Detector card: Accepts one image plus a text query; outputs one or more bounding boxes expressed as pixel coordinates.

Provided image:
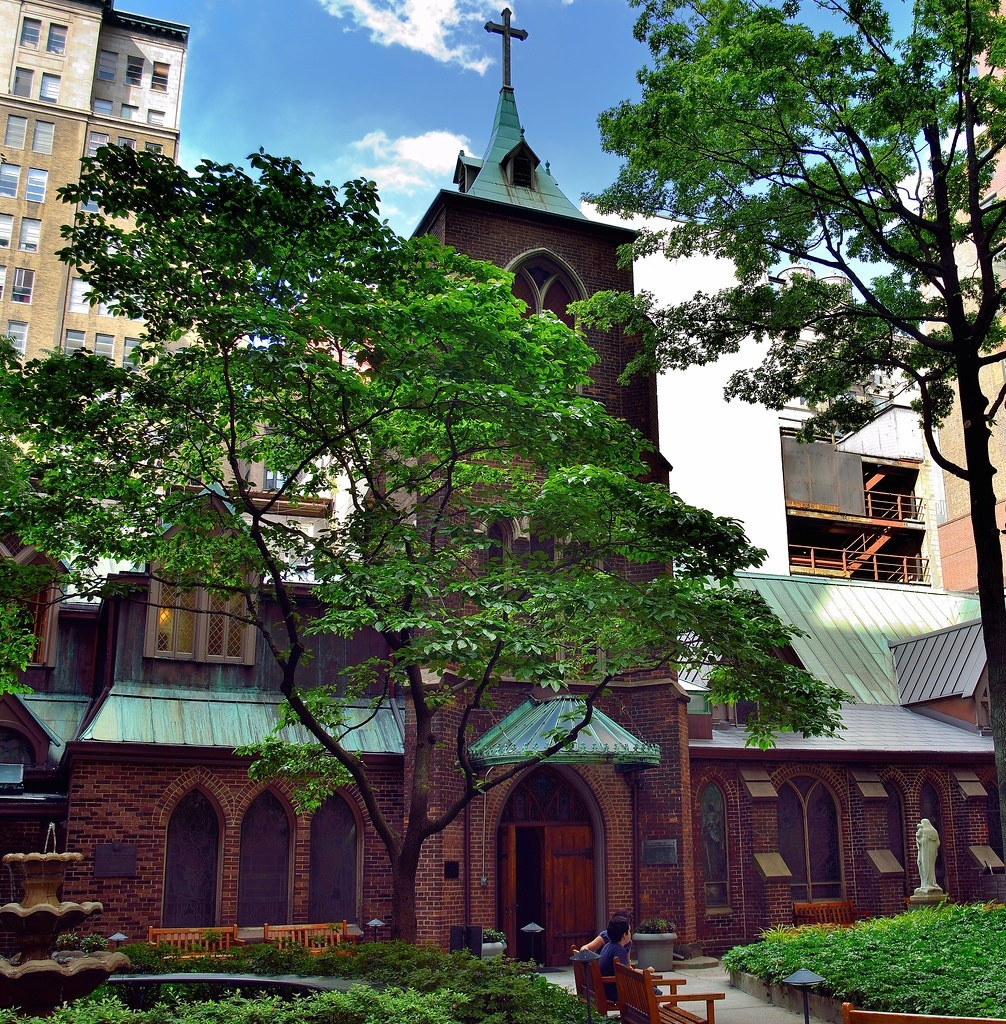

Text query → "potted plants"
[632,918,677,971]
[482,927,507,959]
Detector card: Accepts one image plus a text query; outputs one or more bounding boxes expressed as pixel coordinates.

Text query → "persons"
[581,910,657,987]
[599,915,663,1007]
[916,820,940,889]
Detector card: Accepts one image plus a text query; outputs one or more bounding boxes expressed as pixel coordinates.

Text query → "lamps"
[366,918,386,943]
[782,966,826,1024]
[520,922,544,986]
[0,763,24,795]
[108,932,128,949]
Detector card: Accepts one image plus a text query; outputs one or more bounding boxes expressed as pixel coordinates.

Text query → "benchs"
[842,1003,1006,1024]
[793,899,870,933]
[264,920,356,958]
[572,945,664,1016]
[150,924,245,960]
[613,956,725,1024]
[904,898,956,912]
